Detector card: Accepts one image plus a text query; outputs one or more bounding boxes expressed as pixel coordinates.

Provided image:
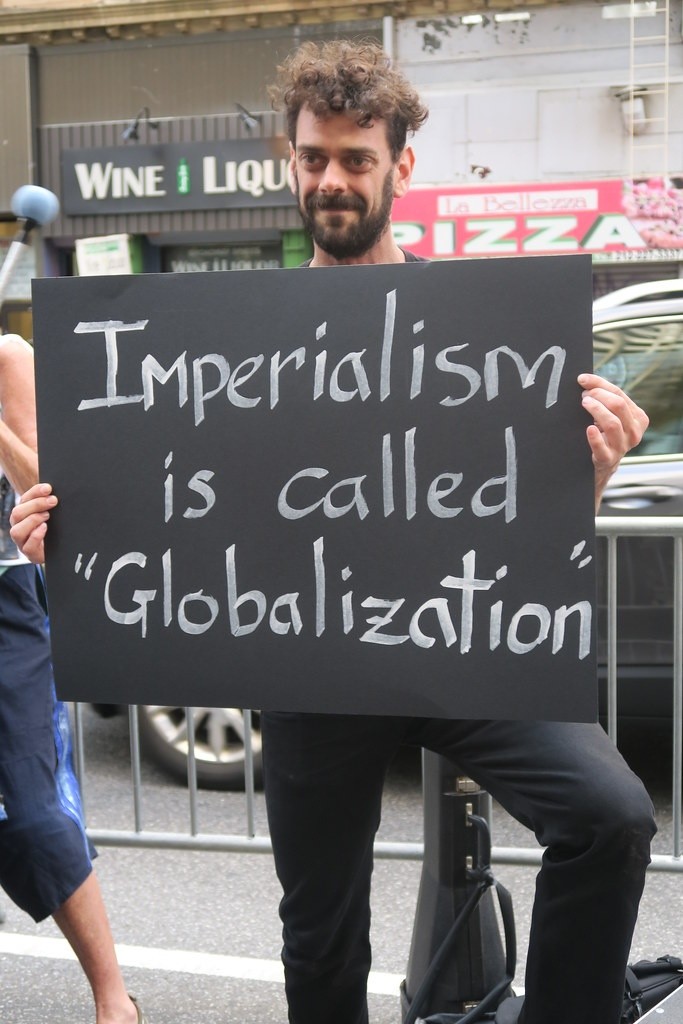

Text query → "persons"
[9,42,658,1024]
[0,333,143,1024]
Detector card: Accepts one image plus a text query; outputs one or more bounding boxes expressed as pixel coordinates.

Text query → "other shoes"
[129,995,148,1024]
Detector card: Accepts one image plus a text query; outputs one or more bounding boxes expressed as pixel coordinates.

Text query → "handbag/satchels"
[621,954,683,1024]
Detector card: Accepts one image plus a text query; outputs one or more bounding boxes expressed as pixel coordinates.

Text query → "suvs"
[90,279,683,791]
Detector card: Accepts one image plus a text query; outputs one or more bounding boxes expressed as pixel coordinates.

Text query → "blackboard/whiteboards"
[28,251,613,728]
